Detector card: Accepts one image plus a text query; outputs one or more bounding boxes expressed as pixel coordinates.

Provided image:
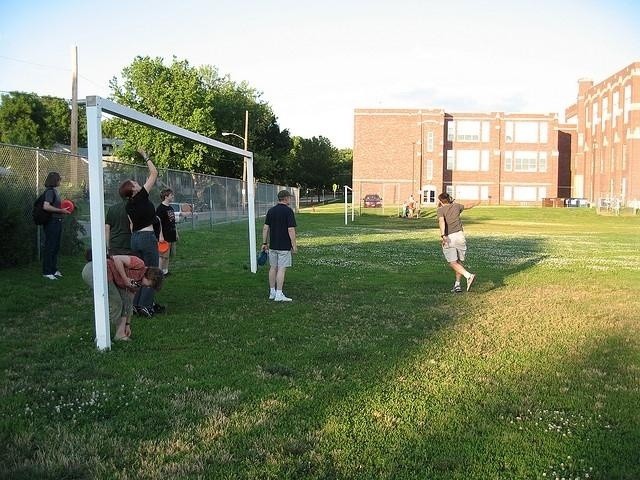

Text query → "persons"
[401,194,421,218]
[105,197,131,255]
[155,188,178,277]
[39,171,72,280]
[436,192,483,292]
[81,253,163,342]
[260,189,299,302]
[119,145,159,317]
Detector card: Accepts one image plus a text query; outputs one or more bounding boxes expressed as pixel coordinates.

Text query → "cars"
[362,193,383,209]
[169,202,199,224]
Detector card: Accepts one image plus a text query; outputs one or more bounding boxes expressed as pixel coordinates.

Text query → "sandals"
[114,335,133,342]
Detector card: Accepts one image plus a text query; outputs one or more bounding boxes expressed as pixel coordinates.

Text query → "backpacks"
[32,188,56,225]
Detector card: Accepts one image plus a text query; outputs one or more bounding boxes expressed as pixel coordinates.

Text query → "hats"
[277,190,295,197]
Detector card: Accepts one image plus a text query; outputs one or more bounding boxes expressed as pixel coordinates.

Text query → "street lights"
[220,130,247,214]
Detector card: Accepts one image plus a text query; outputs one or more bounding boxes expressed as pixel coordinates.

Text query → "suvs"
[179,198,209,212]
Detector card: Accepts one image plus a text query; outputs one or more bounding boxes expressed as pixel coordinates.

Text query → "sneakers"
[451,286,461,293]
[467,274,476,292]
[269,293,293,302]
[139,307,154,318]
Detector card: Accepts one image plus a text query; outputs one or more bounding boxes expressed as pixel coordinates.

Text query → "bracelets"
[441,234,446,238]
[261,242,269,245]
[125,322,130,324]
[143,157,151,164]
